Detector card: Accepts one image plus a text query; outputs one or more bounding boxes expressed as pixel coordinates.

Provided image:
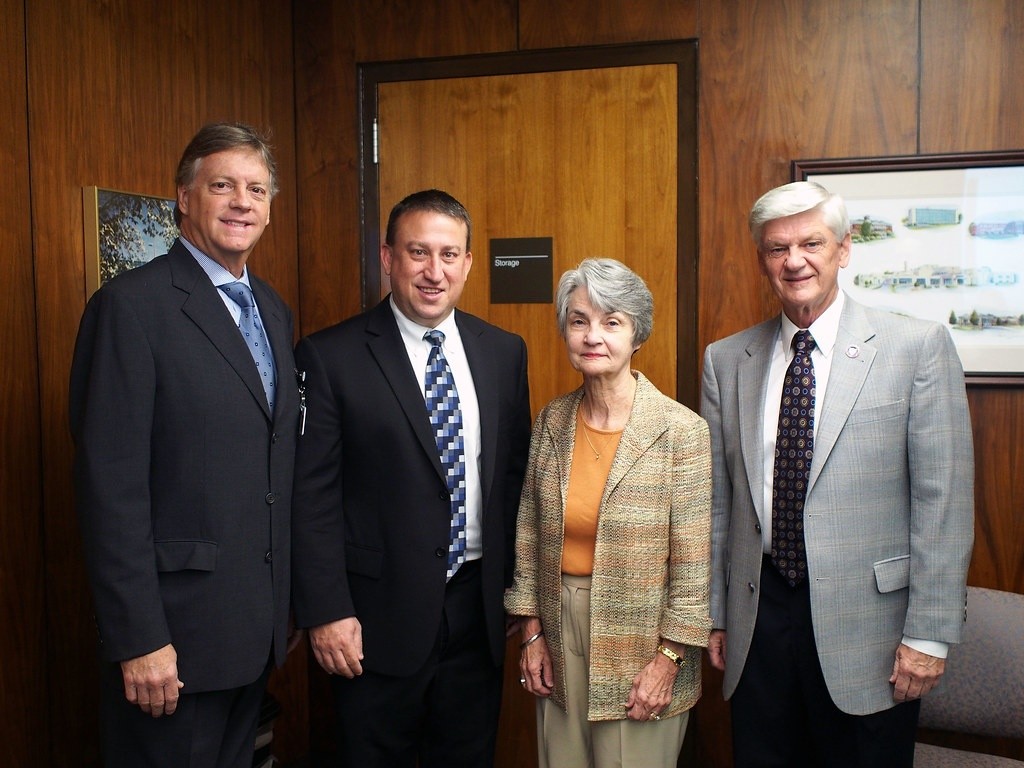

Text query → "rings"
[650,712,660,720]
[521,679,526,683]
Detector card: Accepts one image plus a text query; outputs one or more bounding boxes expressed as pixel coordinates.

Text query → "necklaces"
[582,392,631,461]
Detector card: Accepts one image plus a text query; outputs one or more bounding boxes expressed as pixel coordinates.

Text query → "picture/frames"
[791,150,1024,386]
[83,185,180,303]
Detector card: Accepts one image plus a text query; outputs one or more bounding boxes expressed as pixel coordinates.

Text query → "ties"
[772,329,820,590]
[221,280,278,415]
[423,330,467,586]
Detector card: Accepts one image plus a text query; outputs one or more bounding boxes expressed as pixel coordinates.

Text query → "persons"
[700,179,976,768]
[289,191,533,768]
[66,123,298,768]
[501,257,713,768]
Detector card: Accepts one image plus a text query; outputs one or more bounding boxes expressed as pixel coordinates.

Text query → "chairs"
[911,586,1024,768]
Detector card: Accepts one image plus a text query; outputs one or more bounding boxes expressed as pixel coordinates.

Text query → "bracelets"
[520,629,544,650]
[658,644,686,669]
